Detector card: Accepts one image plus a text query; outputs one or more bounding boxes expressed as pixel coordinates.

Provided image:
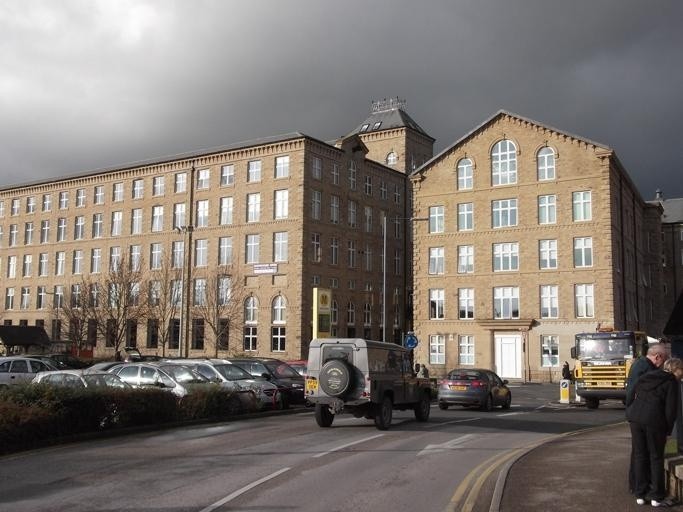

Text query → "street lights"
[174,225,194,357]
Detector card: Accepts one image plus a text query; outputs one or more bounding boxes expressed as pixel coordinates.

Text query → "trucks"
[570,327,662,410]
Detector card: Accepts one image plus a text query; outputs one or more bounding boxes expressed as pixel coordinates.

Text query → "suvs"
[303,338,435,431]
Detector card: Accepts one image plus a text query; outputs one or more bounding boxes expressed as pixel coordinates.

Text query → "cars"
[436,368,512,412]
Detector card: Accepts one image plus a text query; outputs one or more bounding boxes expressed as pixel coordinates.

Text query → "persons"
[562,361,570,378]
[626,345,668,494]
[416,364,429,379]
[625,358,683,508]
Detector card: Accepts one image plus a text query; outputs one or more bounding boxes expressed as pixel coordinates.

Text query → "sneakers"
[636,498,645,505]
[650,499,661,507]
